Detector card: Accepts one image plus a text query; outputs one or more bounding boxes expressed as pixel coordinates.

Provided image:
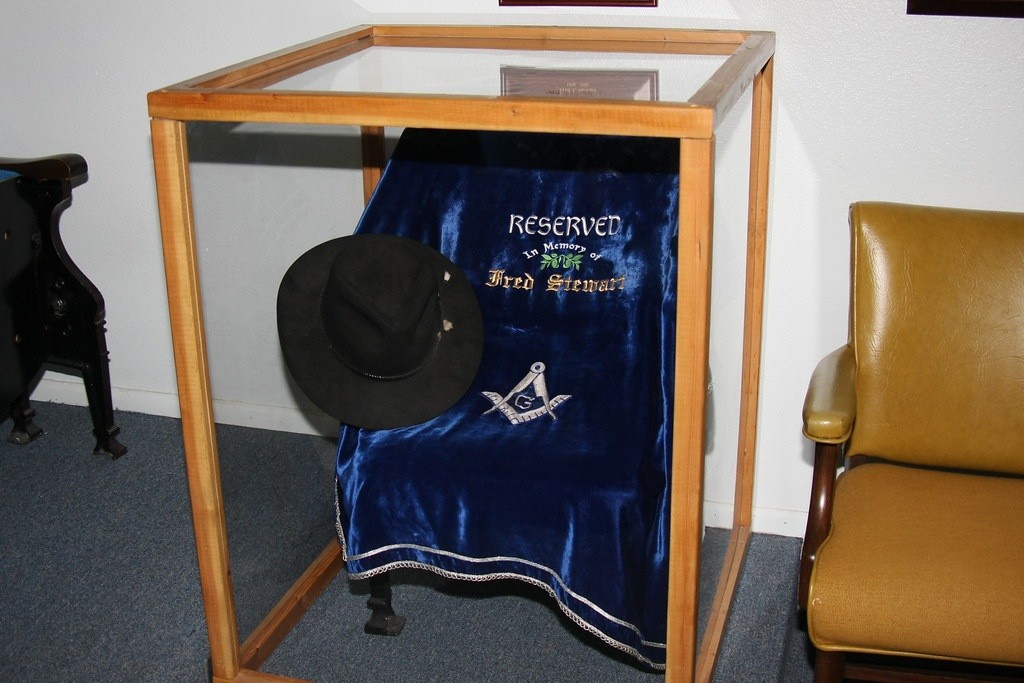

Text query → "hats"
[277,233,482,430]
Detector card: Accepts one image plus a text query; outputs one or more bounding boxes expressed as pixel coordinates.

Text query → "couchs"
[792,200,1024,683]
